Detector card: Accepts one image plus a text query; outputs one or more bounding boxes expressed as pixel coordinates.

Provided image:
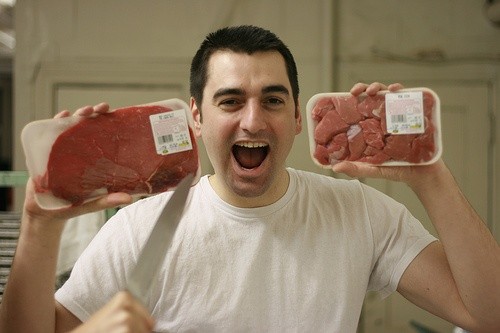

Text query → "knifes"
[124,171,195,305]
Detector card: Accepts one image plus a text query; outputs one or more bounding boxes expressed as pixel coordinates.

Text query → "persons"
[0,25,500,333]
[69,289,156,333]
